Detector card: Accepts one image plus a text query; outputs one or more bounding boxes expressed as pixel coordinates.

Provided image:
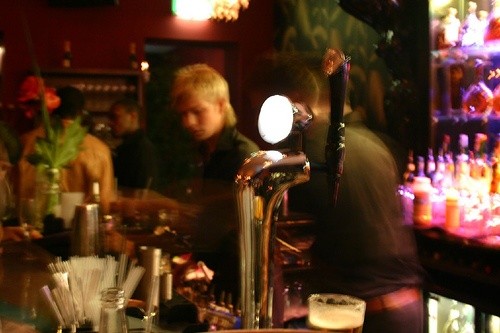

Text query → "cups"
[307,293,367,333]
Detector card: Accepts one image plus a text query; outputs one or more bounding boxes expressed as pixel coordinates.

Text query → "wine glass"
[18,197,46,264]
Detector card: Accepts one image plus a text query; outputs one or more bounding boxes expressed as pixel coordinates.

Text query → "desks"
[410,221,500,333]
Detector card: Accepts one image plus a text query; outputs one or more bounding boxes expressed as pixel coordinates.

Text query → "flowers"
[14,75,93,215]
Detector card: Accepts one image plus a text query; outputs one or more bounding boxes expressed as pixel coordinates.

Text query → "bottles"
[412,176,432,225]
[403,133,499,199]
[445,196,459,227]
[97,287,128,333]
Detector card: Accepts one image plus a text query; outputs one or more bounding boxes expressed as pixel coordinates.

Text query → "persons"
[93,98,167,192]
[110,63,260,308]
[249,47,429,333]
[15,85,115,227]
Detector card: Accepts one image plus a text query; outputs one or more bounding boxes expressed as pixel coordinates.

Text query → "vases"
[34,163,75,238]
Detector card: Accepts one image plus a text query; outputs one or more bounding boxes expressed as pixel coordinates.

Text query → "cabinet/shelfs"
[273,211,314,322]
[425,0,500,149]
[39,65,146,148]
